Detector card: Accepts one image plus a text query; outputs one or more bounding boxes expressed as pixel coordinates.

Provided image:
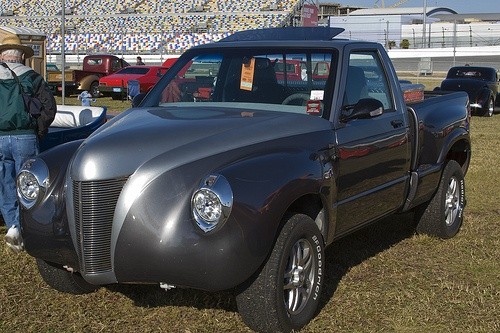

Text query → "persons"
[0,31,57,253]
[136,57,145,66]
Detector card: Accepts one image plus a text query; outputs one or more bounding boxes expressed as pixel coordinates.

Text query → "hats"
[0,33,35,58]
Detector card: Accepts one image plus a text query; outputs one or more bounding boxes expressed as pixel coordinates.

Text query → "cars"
[38,105,108,154]
[434,65,500,117]
[99,57,193,100]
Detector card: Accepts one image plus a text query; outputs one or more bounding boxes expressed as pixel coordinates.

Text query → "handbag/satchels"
[22,94,42,116]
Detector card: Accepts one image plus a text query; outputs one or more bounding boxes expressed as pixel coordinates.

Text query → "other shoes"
[5,225,23,251]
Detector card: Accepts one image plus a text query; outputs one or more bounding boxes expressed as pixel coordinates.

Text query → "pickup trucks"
[14,25,474,333]
[197,59,330,99]
[47,53,124,98]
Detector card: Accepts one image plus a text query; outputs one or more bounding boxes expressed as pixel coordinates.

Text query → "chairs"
[346,65,369,103]
[126,80,142,103]
[222,68,279,105]
